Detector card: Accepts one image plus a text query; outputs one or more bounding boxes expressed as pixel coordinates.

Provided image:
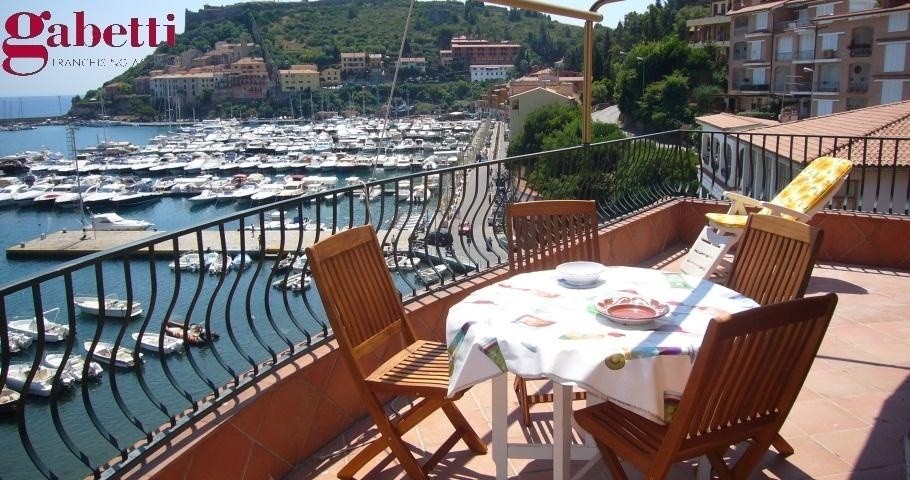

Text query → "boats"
[0,294,220,412]
[169,249,449,292]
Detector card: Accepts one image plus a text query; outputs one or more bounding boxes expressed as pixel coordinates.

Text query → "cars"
[479,119,522,253]
[457,220,472,236]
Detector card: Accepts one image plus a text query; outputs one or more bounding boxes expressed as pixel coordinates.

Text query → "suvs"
[424,230,453,247]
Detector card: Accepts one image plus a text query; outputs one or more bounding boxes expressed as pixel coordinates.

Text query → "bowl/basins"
[558,261,605,285]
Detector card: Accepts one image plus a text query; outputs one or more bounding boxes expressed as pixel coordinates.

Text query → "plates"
[595,296,670,324]
